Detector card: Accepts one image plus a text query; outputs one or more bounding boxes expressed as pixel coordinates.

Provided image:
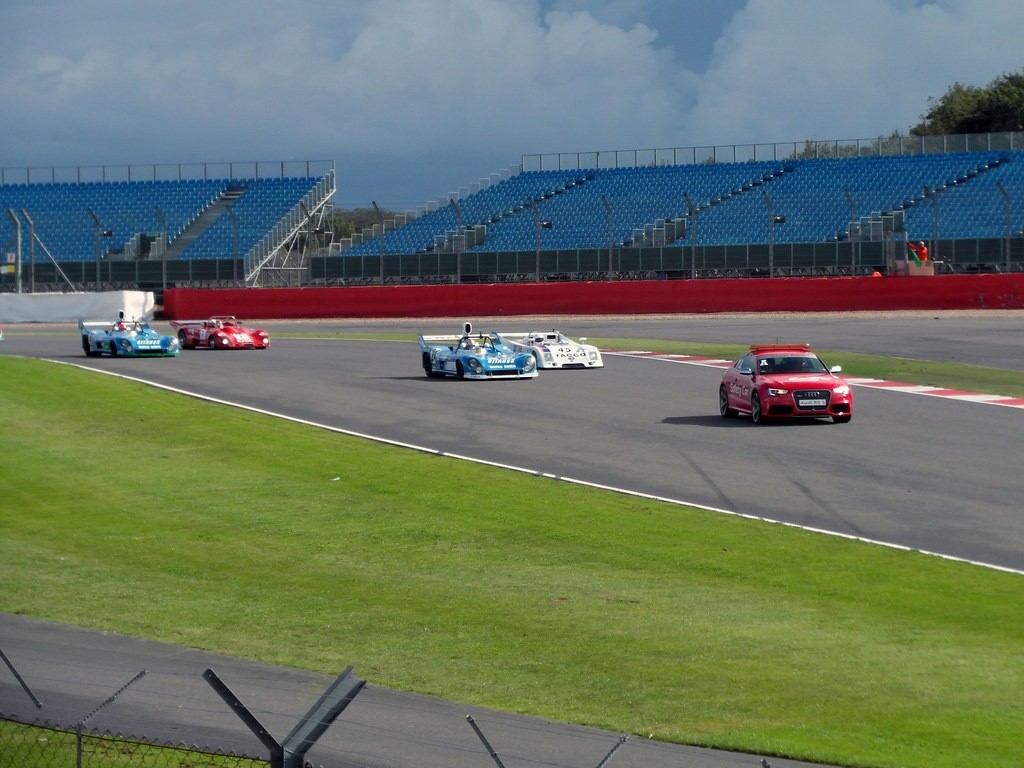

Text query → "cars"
[719,344,853,426]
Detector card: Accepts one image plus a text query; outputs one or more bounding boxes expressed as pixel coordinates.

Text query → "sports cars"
[169,315,270,350]
[417,322,539,382]
[488,327,605,370]
[78,310,179,358]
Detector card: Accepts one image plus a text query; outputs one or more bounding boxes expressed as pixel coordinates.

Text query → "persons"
[215,320,223,328]
[766,358,776,373]
[118,323,127,332]
[463,338,475,350]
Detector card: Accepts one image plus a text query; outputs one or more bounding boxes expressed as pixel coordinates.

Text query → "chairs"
[0,149,1024,266]
